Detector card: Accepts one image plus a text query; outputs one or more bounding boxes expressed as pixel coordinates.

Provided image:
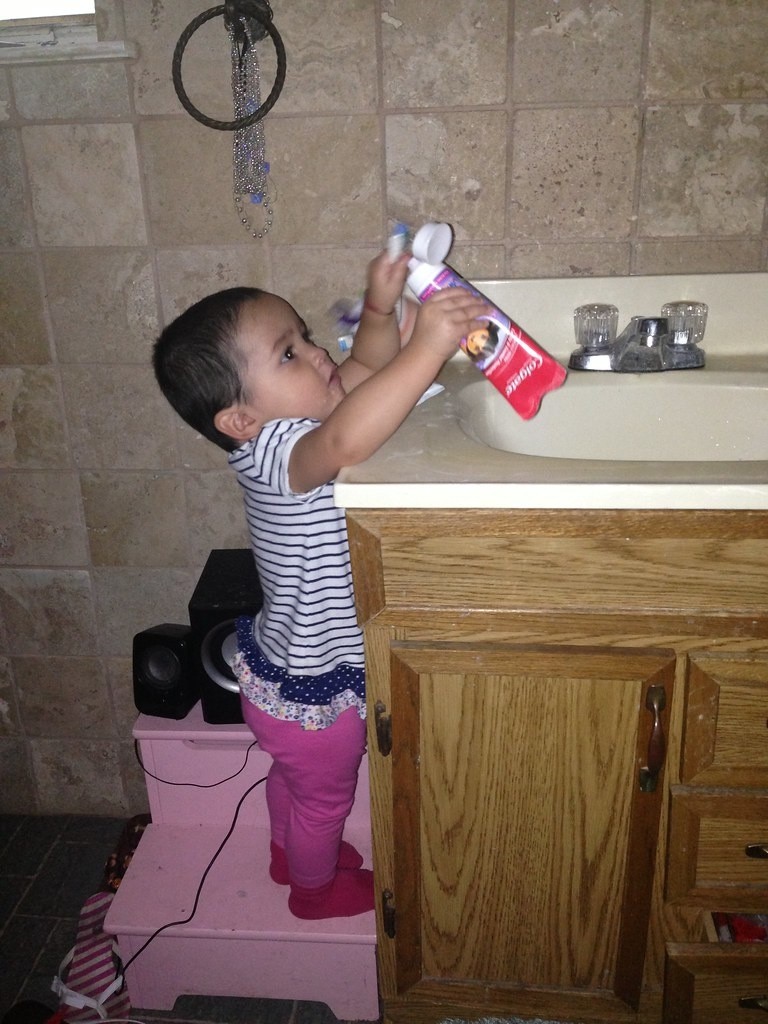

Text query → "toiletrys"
[405,220,571,421]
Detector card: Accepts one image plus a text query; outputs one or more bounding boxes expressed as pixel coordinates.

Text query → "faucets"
[611,315,670,372]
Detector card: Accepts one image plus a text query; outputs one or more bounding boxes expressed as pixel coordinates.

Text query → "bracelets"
[364,288,396,317]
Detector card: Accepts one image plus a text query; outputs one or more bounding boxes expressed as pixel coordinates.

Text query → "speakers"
[131,624,200,719]
[187,549,265,726]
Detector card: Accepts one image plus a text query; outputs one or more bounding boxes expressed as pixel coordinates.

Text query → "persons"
[153,250,488,920]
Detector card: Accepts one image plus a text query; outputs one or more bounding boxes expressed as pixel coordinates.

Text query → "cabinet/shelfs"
[346,504,768,1021]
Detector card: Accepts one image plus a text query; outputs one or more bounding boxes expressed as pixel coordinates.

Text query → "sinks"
[449,355,767,485]
